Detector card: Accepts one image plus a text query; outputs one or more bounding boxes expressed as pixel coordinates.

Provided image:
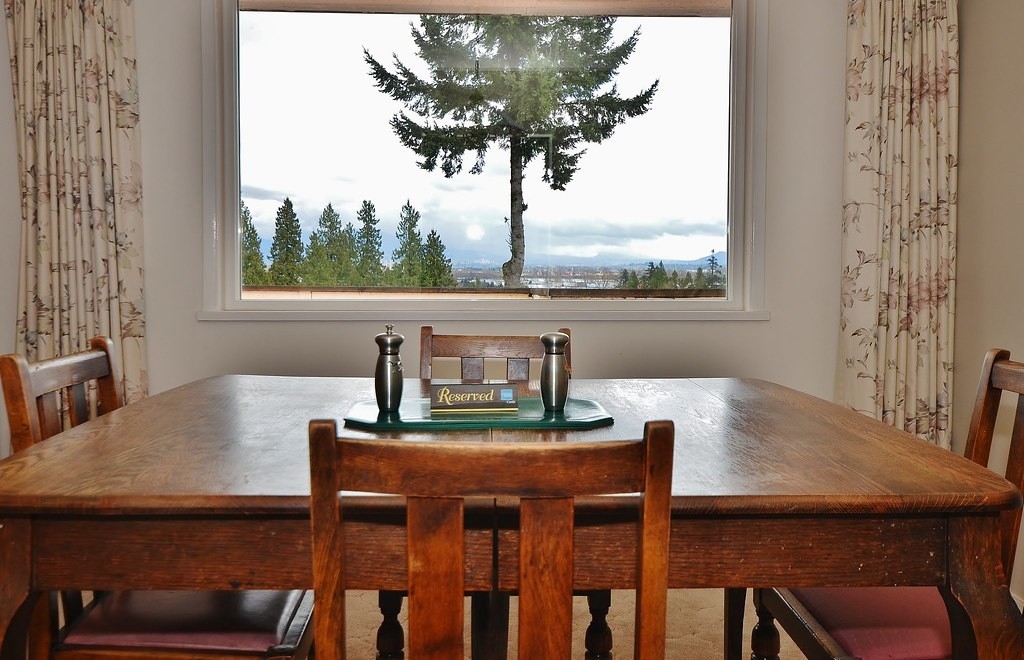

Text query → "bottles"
[541,333,569,415]
[373,325,405,414]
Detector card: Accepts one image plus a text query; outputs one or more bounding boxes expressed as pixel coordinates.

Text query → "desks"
[0,379,1024,660]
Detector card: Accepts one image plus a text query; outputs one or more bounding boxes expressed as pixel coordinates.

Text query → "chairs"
[309,325,675,660]
[750,346,1023,659]
[0,338,316,660]
